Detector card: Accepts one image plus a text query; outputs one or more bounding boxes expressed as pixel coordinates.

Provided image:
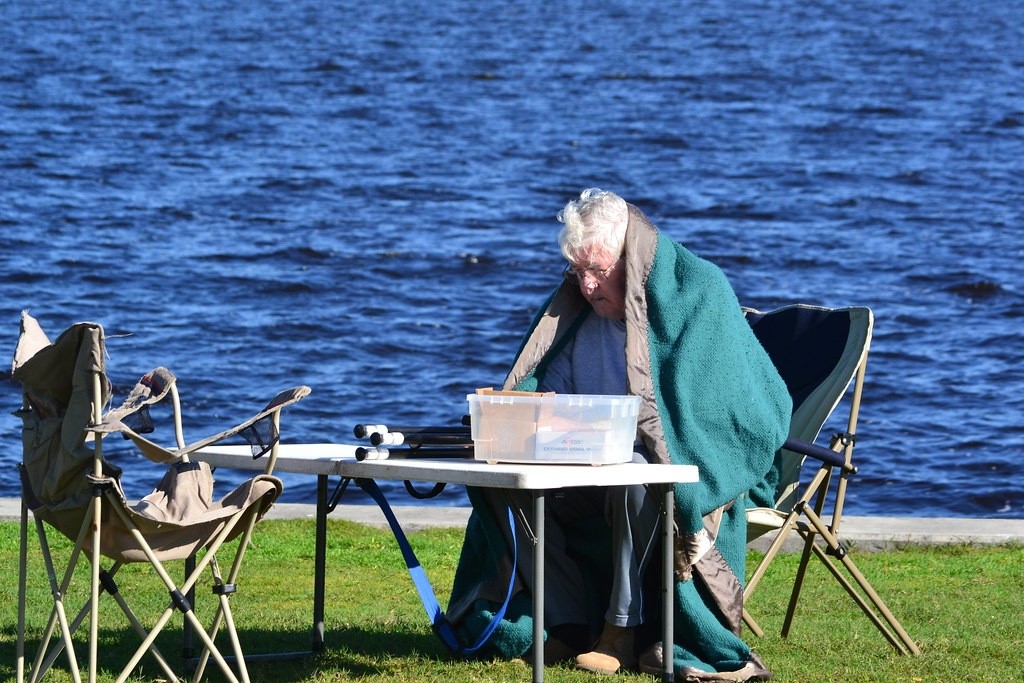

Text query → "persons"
[445,188,791,668]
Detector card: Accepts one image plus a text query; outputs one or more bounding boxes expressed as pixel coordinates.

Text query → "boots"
[574,623,634,675]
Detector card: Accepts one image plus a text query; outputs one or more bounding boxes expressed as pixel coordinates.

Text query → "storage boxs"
[467,392,644,466]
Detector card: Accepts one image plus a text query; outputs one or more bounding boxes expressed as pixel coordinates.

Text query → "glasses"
[562,252,625,283]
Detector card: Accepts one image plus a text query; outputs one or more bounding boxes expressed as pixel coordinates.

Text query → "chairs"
[738,303,924,658]
[10,308,312,683]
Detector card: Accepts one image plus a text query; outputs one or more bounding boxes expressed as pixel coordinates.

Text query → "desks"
[169,441,699,683]
